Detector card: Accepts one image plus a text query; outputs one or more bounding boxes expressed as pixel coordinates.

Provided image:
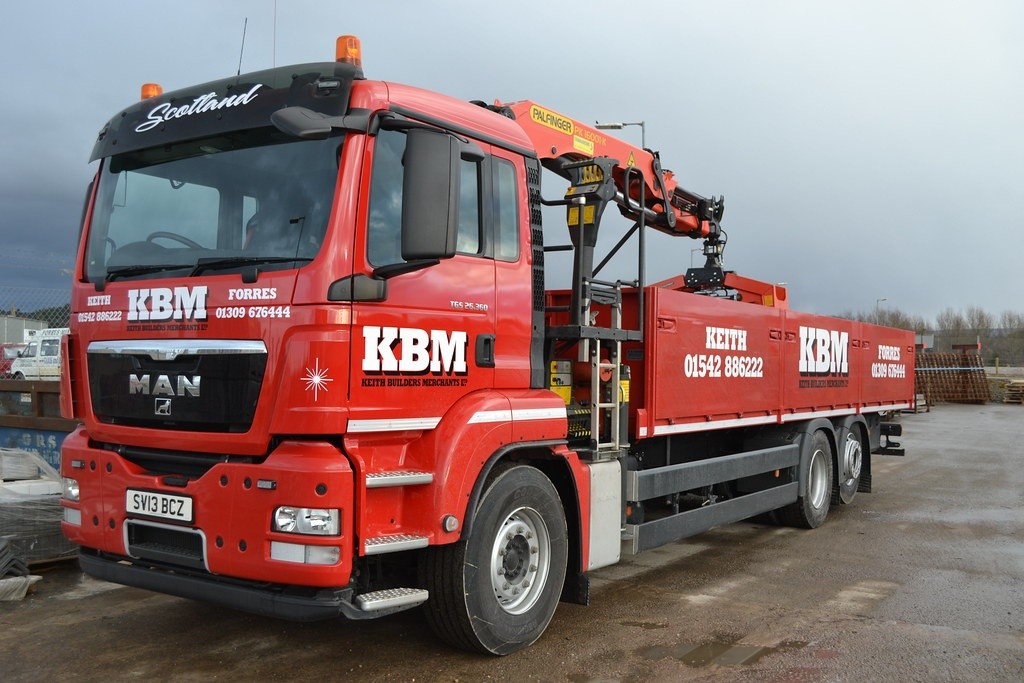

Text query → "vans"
[11,327,72,382]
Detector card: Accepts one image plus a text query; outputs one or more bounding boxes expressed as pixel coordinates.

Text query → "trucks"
[60,34,917,657]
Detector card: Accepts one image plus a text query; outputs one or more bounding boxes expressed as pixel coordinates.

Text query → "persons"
[241,185,320,259]
